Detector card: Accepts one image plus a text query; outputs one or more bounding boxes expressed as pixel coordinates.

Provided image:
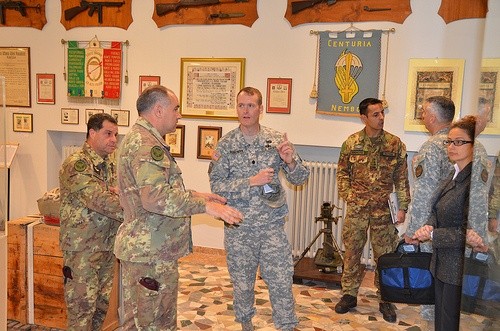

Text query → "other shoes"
[335,294,357,313]
[379,303,396,323]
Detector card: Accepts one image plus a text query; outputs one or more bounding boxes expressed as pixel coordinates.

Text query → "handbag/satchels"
[460,247,486,312]
[376,239,438,305]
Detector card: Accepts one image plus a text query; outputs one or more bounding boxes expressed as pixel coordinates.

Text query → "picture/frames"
[1,47,466,160]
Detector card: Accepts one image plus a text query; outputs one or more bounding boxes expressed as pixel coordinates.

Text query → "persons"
[208,87,310,331]
[412,115,477,331]
[59,113,124,331]
[404,96,462,321]
[113,84,245,331]
[465,97,500,331]
[335,98,411,322]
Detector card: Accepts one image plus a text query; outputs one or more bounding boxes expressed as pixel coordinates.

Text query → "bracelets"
[431,230,433,241]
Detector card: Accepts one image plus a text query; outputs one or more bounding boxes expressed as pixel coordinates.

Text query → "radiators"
[292,160,348,257]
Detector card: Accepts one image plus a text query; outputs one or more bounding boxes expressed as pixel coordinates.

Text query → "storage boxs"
[7,215,122,331]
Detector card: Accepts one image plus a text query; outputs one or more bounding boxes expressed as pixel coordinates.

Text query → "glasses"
[443,140,472,146]
